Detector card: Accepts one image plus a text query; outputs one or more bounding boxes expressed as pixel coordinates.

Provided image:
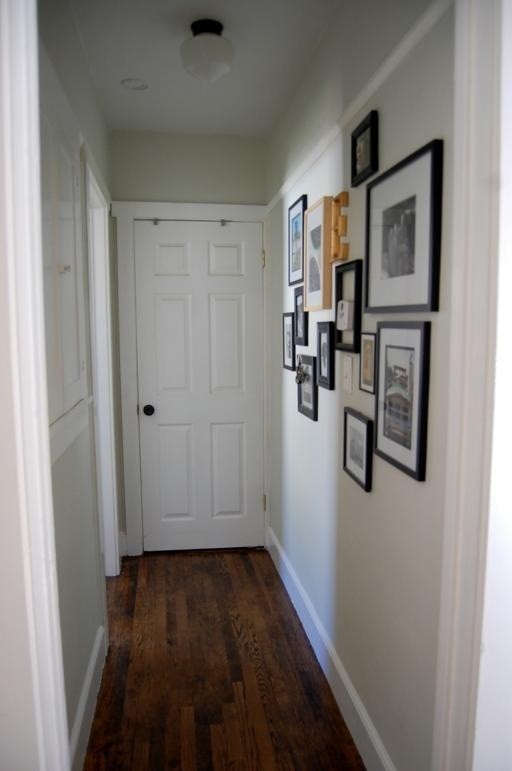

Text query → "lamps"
[179,15,236,85]
